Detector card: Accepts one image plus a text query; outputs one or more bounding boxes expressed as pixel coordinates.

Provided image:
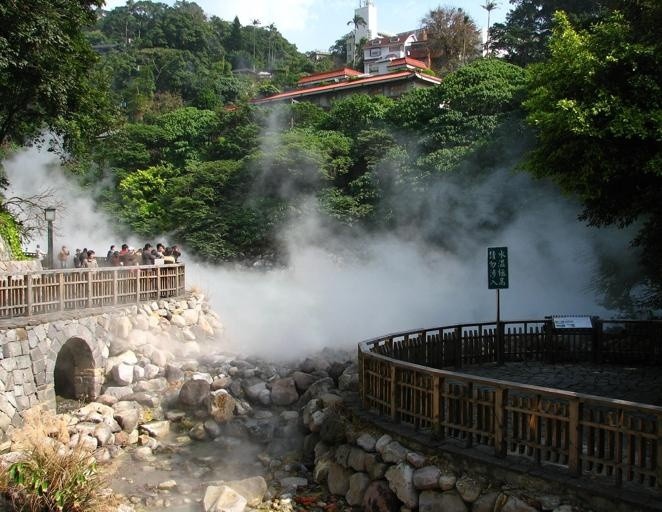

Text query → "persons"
[33,238,184,300]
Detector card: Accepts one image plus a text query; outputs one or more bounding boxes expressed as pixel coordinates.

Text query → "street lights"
[43,204,56,270]
